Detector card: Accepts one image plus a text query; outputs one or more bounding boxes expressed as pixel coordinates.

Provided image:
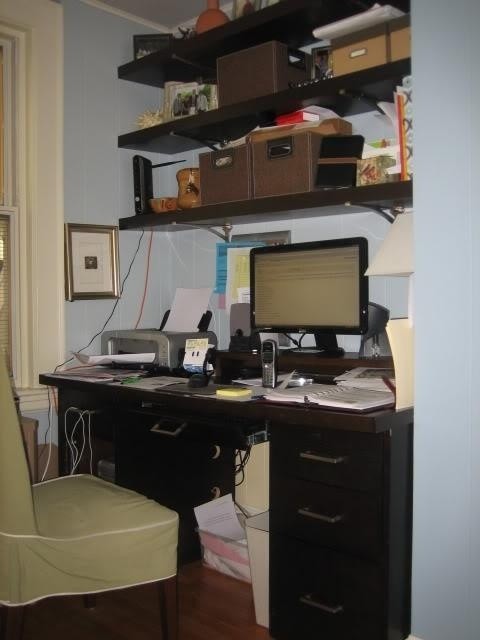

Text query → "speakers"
[230,303,261,354]
[359,302,393,359]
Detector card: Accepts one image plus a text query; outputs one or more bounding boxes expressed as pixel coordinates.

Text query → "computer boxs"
[114,416,236,567]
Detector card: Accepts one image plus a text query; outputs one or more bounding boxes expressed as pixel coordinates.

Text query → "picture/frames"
[311,45,334,83]
[64,223,121,301]
[133,34,173,60]
[164,76,218,124]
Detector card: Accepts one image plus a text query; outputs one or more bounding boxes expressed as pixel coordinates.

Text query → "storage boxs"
[331,14,411,77]
[251,130,324,200]
[195,513,252,585]
[216,40,312,111]
[198,144,252,206]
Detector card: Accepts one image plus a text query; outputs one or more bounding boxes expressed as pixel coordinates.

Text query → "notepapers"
[216,388,252,398]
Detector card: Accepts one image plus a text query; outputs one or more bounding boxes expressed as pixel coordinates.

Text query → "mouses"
[188,373,209,388]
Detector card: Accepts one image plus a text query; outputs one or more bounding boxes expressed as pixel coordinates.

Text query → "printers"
[101,309,218,375]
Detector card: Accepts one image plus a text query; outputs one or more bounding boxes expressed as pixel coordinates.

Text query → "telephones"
[262,339,277,389]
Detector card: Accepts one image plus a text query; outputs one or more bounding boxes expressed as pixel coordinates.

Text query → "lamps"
[364,213,414,409]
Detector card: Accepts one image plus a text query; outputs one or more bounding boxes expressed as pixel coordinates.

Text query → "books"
[264,383,396,414]
[49,366,189,391]
[215,105,365,188]
[312,5,405,40]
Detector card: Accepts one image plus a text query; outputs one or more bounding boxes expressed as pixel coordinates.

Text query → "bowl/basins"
[148,196,177,214]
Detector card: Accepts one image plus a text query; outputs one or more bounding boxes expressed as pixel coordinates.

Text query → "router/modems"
[132,154,187,215]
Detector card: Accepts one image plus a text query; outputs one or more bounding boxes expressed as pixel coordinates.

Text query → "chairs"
[0,355,181,640]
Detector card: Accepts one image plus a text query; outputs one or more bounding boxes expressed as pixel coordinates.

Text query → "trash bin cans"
[245,510,269,629]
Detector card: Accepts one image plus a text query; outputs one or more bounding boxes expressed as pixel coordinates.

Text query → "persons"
[136,48,152,59]
[177,26,197,40]
[173,89,209,116]
[243,0,252,16]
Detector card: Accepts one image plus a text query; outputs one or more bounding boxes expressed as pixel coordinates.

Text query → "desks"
[39,350,414,640]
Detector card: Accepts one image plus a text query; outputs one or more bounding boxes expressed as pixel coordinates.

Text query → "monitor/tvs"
[249,237,369,359]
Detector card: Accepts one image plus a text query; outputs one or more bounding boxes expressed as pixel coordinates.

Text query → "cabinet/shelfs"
[118,0,413,244]
